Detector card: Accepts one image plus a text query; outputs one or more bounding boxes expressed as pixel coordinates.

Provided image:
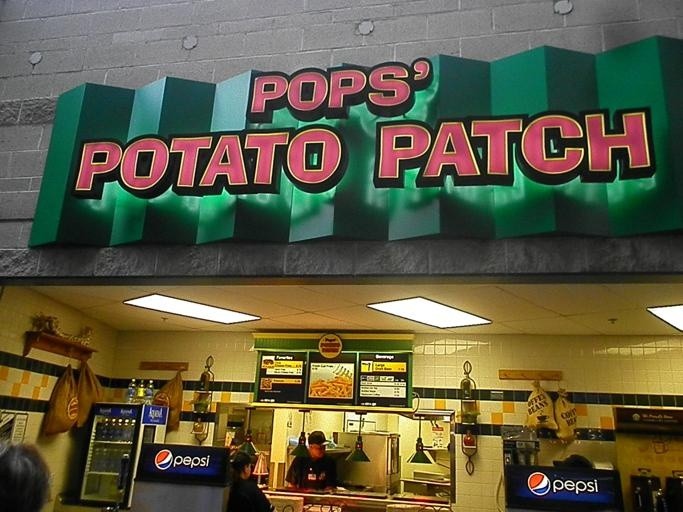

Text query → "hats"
[308,431,332,447]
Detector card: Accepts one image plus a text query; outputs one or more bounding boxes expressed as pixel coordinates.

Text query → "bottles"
[89,446,131,473]
[93,417,136,442]
[126,378,153,406]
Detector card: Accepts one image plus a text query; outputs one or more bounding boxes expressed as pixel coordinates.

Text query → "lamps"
[239,407,435,465]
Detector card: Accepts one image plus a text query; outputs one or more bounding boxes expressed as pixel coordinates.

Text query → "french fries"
[309,377,352,397]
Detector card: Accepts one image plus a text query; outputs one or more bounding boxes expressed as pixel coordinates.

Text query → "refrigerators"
[68,401,169,506]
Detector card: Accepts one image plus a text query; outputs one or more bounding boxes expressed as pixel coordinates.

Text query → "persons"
[285,429,338,492]
[225,452,278,512]
[0,440,52,512]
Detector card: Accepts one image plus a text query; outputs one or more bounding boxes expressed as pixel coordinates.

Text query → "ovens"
[333,432,401,495]
